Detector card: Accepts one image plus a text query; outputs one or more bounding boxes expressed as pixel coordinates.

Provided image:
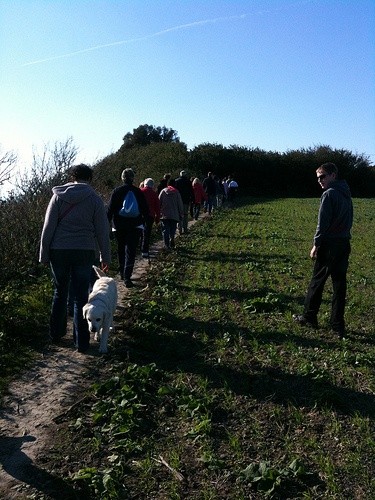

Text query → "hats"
[180,170,187,177]
[144,178,154,187]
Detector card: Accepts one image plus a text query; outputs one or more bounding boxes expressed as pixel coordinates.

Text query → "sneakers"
[291,314,318,326]
[328,321,345,331]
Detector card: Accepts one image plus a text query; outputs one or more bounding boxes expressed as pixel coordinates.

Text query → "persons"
[107,163,238,351]
[292,163,353,336]
[38,164,112,358]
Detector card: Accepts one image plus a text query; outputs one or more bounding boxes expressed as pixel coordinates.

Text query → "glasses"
[316,172,332,180]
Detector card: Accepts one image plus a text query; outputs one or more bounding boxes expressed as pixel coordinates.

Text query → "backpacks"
[117,186,140,217]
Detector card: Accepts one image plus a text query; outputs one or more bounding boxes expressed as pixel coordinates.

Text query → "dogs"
[82,265,118,355]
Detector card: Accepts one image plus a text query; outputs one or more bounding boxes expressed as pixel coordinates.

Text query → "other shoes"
[119,273,123,280]
[50,328,66,337]
[73,339,90,353]
[124,279,133,287]
